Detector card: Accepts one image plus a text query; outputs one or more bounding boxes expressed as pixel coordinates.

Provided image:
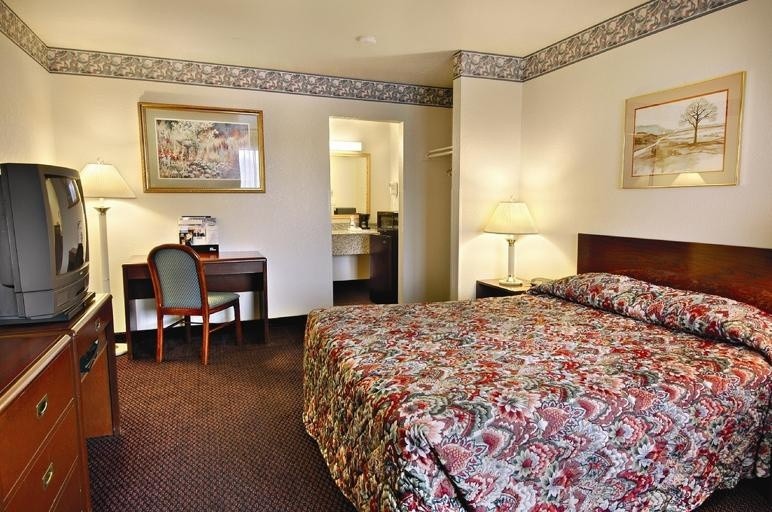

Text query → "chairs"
[146,244,242,365]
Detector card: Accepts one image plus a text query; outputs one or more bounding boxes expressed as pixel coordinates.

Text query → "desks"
[1,293,121,511]
[122,251,268,359]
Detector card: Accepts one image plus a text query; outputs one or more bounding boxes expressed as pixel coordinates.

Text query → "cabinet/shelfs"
[369,234,398,305]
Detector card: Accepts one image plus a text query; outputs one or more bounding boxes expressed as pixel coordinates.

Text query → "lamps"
[79,158,137,358]
[483,196,540,286]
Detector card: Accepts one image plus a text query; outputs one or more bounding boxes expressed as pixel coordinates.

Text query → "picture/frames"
[138,102,266,194]
[619,70,747,189]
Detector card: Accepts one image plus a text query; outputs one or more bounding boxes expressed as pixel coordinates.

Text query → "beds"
[309,232,770,510]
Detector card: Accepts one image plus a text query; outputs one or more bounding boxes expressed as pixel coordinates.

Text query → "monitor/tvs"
[0,162,95,324]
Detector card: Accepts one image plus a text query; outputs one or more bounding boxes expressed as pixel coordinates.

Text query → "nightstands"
[476,278,544,298]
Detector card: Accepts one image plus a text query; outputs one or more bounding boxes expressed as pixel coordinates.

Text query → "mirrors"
[330,151,371,220]
